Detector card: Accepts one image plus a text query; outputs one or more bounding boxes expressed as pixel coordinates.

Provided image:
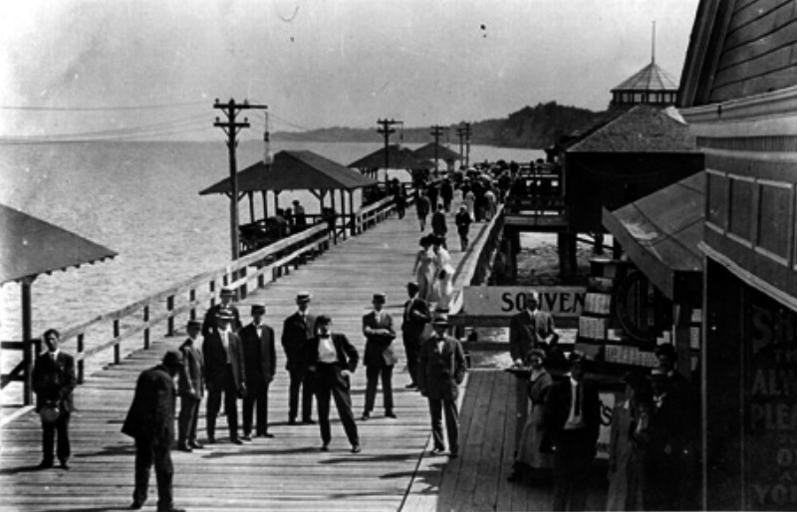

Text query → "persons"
[359,292,398,419]
[276,200,307,233]
[509,343,694,512]
[121,349,186,512]
[201,286,241,333]
[411,235,455,314]
[393,155,563,251]
[179,319,206,450]
[297,314,361,452]
[235,304,276,439]
[281,290,317,425]
[31,330,74,469]
[509,291,556,366]
[400,280,431,392]
[204,309,246,445]
[419,315,468,458]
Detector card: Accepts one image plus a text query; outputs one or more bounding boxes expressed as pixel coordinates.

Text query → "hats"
[162,234,450,365]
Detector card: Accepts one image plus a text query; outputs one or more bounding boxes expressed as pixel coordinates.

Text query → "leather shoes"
[178,382,457,458]
